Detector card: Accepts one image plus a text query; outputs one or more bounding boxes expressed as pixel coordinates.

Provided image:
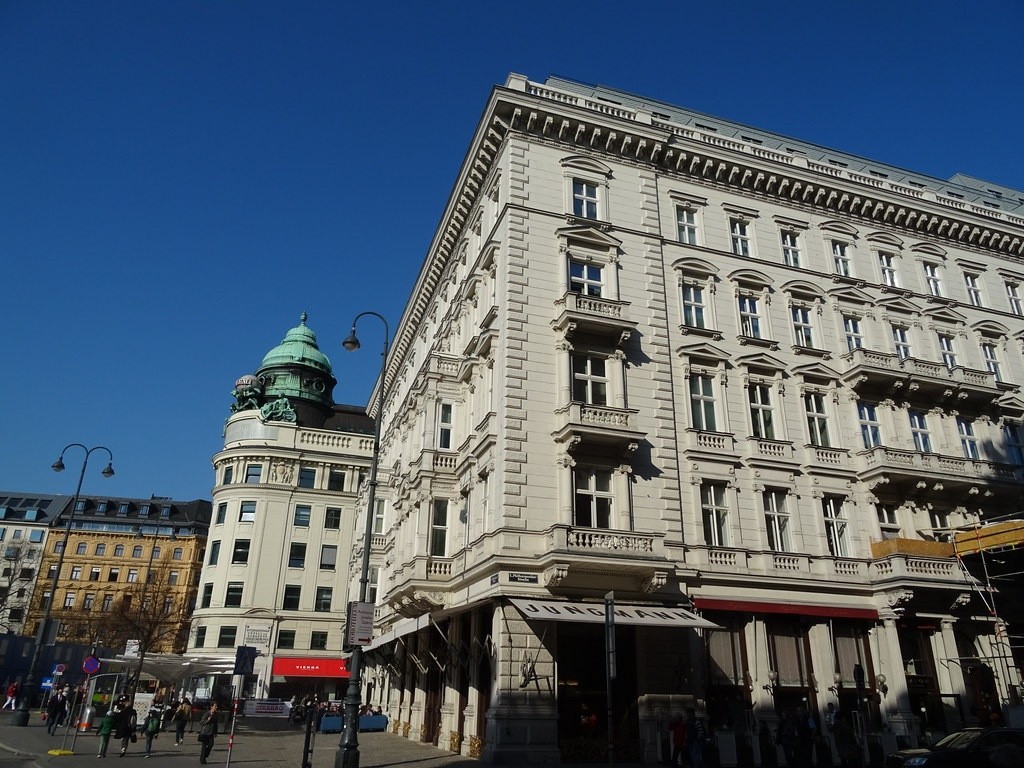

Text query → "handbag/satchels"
[41,715,46,720]
[130,732,137,743]
[198,733,203,742]
[173,703,184,721]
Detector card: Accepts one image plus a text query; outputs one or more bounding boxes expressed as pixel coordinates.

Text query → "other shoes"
[145,756,149,758]
[175,743,180,746]
[97,754,101,758]
[119,748,126,757]
[200,756,207,764]
[179,739,183,745]
[50,732,54,736]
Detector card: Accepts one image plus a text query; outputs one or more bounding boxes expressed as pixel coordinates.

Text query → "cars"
[885,725,1024,768]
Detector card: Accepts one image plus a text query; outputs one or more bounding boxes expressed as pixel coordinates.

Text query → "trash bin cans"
[714,731,741,768]
[78,706,96,733]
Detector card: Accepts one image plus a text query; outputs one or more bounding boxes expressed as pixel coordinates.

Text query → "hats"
[106,710,113,717]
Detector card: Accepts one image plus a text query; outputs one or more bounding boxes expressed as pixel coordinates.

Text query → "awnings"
[271,658,352,678]
[508,598,726,628]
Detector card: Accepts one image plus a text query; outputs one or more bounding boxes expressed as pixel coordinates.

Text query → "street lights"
[9,443,116,727]
[120,514,181,697]
[333,310,392,768]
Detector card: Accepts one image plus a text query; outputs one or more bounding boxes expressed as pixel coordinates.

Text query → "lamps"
[828,673,843,699]
[876,675,887,694]
[763,671,779,698]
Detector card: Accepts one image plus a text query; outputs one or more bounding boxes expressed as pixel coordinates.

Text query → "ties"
[59,695,61,702]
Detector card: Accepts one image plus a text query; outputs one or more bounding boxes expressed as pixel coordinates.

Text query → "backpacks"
[147,716,158,732]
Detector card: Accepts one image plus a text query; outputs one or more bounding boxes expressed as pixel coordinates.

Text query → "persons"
[287,696,382,730]
[1,681,219,764]
[574,702,868,768]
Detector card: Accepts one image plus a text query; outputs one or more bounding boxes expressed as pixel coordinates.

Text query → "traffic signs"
[346,600,375,646]
